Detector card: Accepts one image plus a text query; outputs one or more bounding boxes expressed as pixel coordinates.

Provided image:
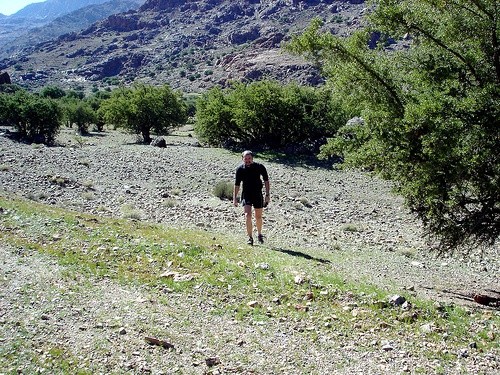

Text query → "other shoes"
[257,234,264,244]
[247,237,253,246]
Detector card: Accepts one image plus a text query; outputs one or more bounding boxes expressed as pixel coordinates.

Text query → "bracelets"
[266,193,269,196]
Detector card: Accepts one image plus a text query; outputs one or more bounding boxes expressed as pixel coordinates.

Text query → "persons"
[233,151,270,245]
